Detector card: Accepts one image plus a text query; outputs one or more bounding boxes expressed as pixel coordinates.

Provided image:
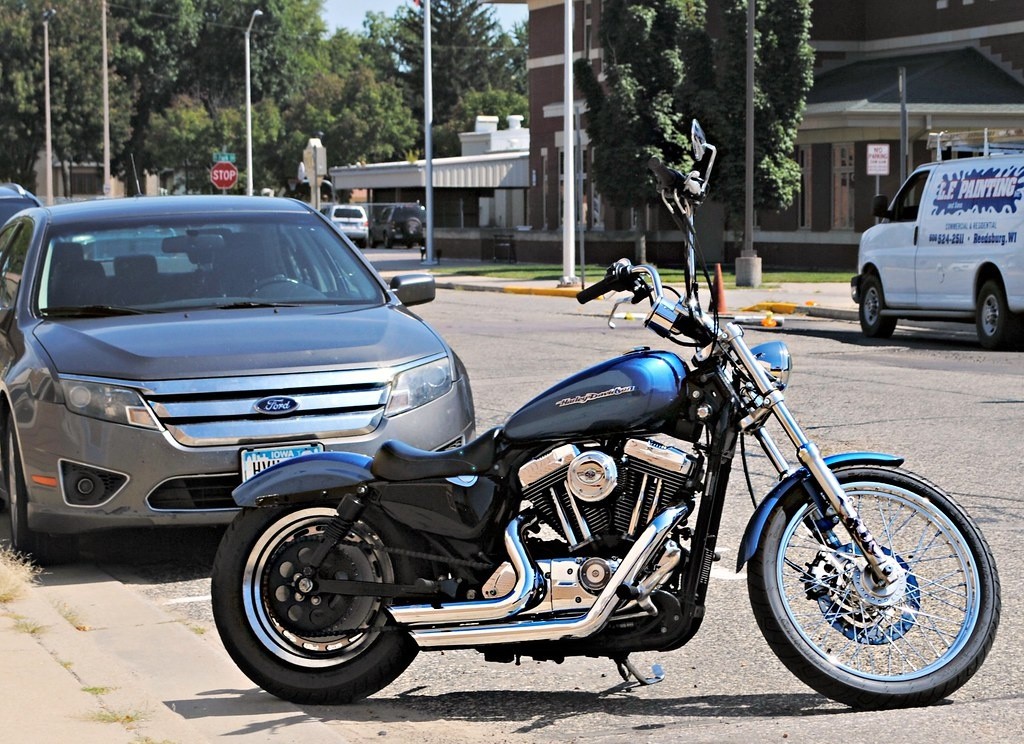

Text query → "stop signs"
[210,161,238,189]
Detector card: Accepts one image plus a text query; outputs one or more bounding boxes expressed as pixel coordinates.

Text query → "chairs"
[49,227,266,307]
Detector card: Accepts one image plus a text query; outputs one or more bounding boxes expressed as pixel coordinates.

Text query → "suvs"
[365,201,426,248]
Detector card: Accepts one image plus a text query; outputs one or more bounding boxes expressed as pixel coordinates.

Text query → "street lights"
[244,9,265,196]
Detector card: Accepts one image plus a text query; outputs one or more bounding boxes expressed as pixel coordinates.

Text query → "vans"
[849,152,1024,351]
[323,204,370,249]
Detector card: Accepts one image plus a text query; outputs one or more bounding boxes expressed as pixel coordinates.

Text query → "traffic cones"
[705,263,734,315]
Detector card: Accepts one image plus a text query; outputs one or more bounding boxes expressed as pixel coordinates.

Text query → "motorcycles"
[208,116,1004,714]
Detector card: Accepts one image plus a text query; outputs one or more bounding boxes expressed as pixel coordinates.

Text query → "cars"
[0,192,476,572]
[0,182,43,230]
